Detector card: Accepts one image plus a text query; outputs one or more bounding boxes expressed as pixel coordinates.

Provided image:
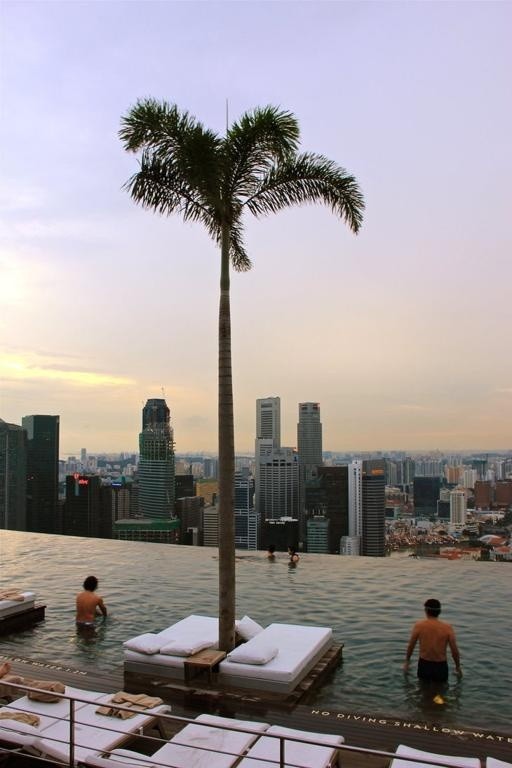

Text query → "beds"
[213,621,337,695]
[118,613,249,684]
[0,588,38,620]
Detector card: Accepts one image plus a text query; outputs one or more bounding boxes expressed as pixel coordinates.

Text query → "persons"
[401,598,463,683]
[288,546,299,562]
[267,544,276,558]
[75,576,107,625]
[0,661,12,679]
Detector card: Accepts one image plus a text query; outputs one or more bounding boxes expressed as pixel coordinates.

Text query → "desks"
[182,648,225,687]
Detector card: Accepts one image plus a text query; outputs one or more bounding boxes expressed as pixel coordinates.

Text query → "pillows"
[121,632,179,655]
[157,636,215,658]
[226,642,281,666]
[232,615,266,642]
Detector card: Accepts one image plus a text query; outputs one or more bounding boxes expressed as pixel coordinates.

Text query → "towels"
[0,588,26,602]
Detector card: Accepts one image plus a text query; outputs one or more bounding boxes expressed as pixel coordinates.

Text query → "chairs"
[237,725,344,766]
[86,713,271,767]
[486,756,510,766]
[1,684,173,767]
[392,744,480,766]
